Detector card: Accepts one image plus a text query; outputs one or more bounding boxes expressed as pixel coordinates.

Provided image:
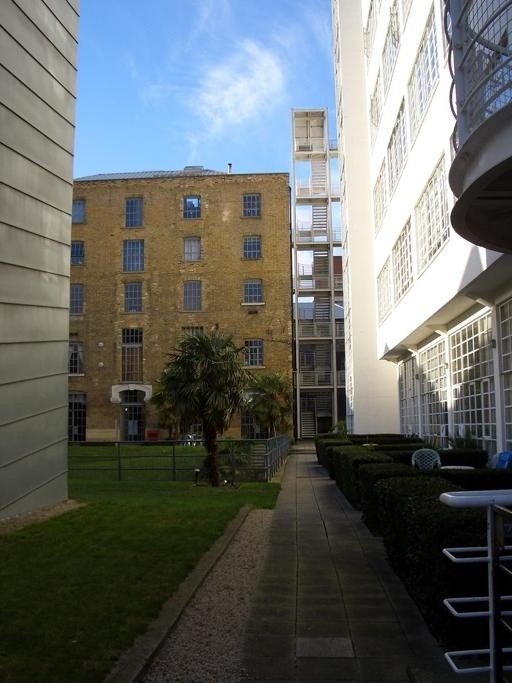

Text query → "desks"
[440,466,474,471]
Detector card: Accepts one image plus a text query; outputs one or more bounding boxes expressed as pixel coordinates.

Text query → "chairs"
[496,451,512,469]
[412,449,441,470]
